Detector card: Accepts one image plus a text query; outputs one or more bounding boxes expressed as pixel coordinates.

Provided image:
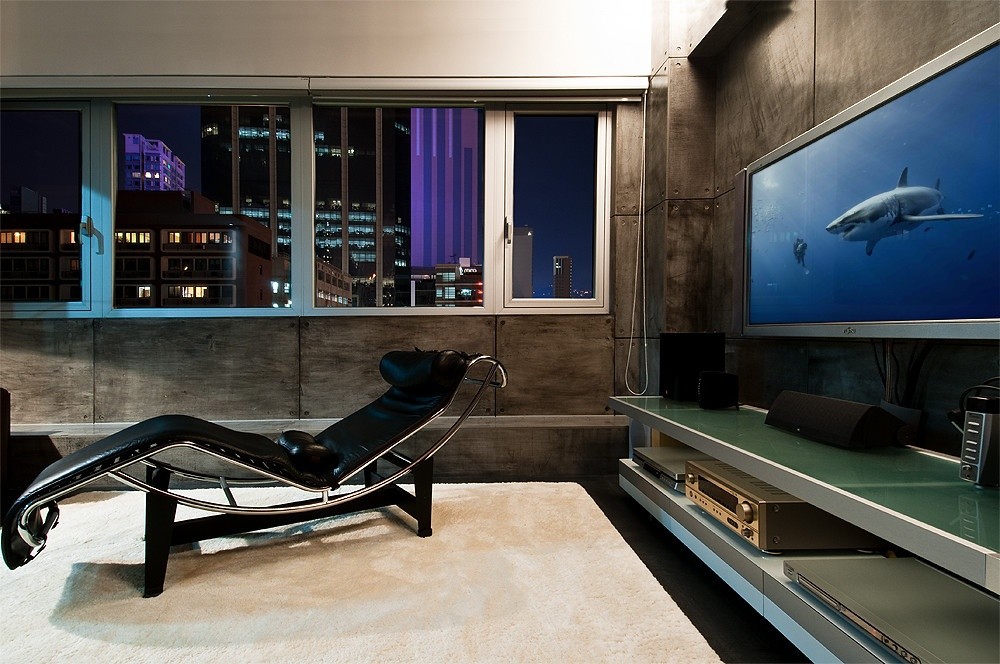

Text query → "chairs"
[0,347,507,598]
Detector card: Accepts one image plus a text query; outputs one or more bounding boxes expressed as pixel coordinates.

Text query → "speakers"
[658,332,726,403]
[959,410,1000,488]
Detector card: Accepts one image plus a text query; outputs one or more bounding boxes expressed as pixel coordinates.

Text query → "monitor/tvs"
[740,23,1000,338]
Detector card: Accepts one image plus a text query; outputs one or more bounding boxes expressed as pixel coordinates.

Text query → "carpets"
[0,482,724,664]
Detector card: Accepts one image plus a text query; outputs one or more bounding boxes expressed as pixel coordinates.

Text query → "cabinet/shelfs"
[608,394,1000,664]
